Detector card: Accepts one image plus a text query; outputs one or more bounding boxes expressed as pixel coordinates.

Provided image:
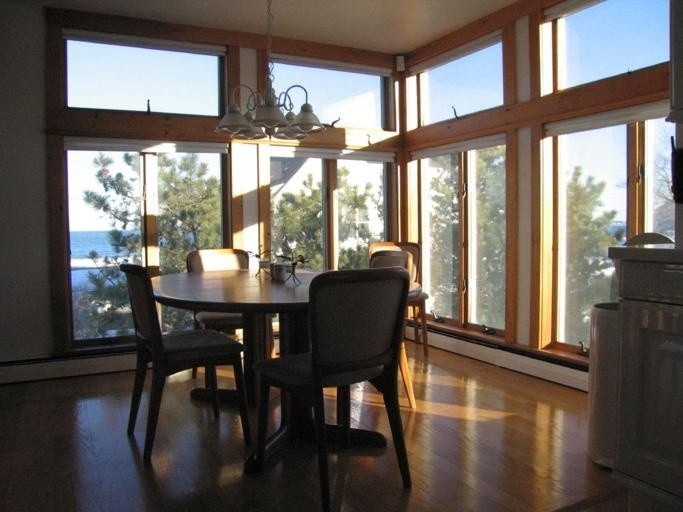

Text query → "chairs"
[367,244,430,358]
[369,251,417,411]
[116,262,254,471]
[185,247,252,377]
[251,268,413,511]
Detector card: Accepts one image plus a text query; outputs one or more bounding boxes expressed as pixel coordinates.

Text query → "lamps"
[213,3,326,147]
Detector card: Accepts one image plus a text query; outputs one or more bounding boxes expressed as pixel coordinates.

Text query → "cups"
[275,264,286,281]
[270,264,278,279]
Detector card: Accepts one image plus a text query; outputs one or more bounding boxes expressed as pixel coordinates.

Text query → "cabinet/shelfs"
[606,240,681,510]
